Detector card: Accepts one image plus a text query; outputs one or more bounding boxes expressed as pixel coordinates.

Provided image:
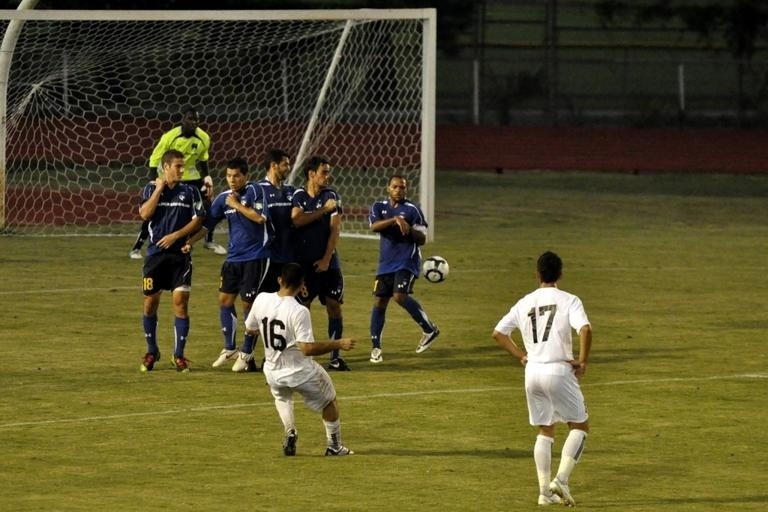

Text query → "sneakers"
[329,356,351,371]
[138,351,159,371]
[212,348,257,373]
[283,428,297,456]
[201,241,227,255]
[416,327,439,353]
[370,348,383,363]
[325,446,354,455]
[171,355,189,373]
[129,249,142,258]
[537,480,576,508]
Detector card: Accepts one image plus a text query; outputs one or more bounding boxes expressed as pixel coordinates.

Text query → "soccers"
[423,256,449,283]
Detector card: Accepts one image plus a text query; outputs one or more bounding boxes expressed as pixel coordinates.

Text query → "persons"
[368,174,440,364]
[181,157,275,372]
[290,156,352,371]
[244,262,356,456]
[130,103,228,260]
[244,148,305,373]
[492,253,592,506]
[138,148,207,372]
